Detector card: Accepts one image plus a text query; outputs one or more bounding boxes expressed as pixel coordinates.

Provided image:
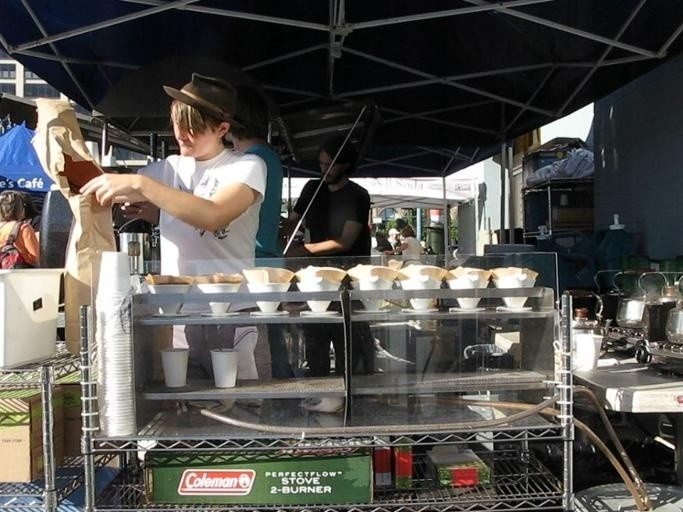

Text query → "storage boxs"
[65,383,97,457]
[1,386,65,485]
[1,267,63,367]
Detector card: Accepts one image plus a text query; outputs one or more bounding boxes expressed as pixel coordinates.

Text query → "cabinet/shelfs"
[0,340,129,512]
[82,254,577,511]
[520,149,596,247]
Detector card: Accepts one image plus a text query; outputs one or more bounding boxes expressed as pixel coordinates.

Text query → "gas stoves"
[635,339,682,374]
[605,327,650,357]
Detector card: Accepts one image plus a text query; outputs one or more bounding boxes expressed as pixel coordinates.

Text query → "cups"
[159,348,189,388]
[574,334,604,372]
[209,348,240,388]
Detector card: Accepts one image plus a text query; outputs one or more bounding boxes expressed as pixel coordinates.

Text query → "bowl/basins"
[146,284,194,314]
[246,282,292,313]
[492,277,536,308]
[196,283,242,313]
[294,282,342,313]
[400,280,443,310]
[445,278,490,309]
[349,280,394,311]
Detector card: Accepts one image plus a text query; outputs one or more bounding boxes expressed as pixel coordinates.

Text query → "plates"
[495,306,532,311]
[250,310,289,315]
[300,310,339,316]
[449,307,487,311]
[353,309,392,314]
[200,312,240,317]
[402,308,439,313]
[151,313,190,317]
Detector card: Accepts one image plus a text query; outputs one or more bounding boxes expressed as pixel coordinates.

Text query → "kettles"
[637,271,680,342]
[593,267,624,321]
[562,288,614,348]
[610,269,654,330]
[665,273,683,345]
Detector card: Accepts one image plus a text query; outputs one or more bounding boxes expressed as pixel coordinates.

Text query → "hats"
[163,72,245,130]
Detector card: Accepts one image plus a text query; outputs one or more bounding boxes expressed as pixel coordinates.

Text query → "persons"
[388,218,407,255]
[79,74,267,379]
[384,224,423,256]
[284,134,377,373]
[376,220,395,256]
[219,86,300,409]
[0,190,40,267]
